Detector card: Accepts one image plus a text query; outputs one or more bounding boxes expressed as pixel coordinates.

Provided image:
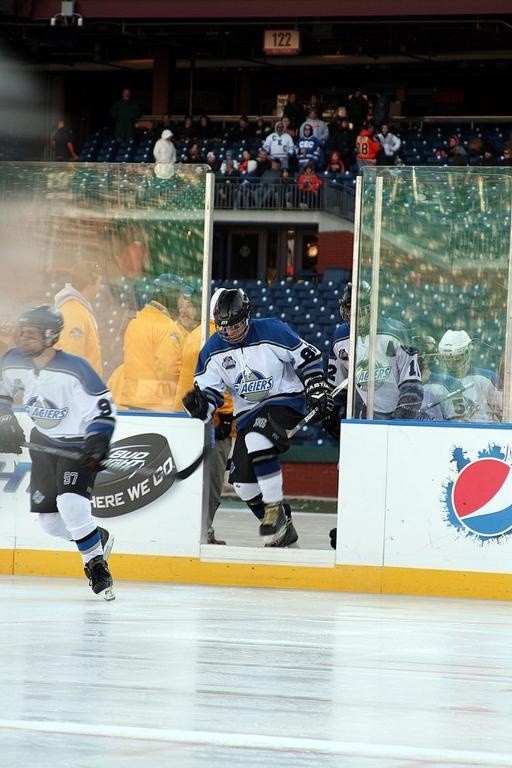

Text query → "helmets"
[210,287,251,344]
[437,328,474,375]
[14,304,63,358]
[339,280,379,334]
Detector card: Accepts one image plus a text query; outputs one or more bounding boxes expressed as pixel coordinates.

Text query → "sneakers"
[83,526,114,593]
[253,500,299,548]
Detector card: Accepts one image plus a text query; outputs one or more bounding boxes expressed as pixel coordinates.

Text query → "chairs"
[43,123,512,381]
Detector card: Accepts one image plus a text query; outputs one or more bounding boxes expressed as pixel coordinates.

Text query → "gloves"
[181,387,210,420]
[1,401,26,454]
[304,382,335,425]
[79,432,110,472]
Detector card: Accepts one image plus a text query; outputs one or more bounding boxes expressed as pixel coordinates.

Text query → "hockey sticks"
[267,359,367,438]
[17,442,208,481]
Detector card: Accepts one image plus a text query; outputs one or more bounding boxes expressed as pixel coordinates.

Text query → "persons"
[49,120,79,162]
[174,292,237,546]
[326,277,503,550]
[1,259,201,411]
[0,304,115,600]
[447,135,512,166]
[222,242,258,279]
[181,287,334,548]
[111,89,135,137]
[154,87,402,210]
